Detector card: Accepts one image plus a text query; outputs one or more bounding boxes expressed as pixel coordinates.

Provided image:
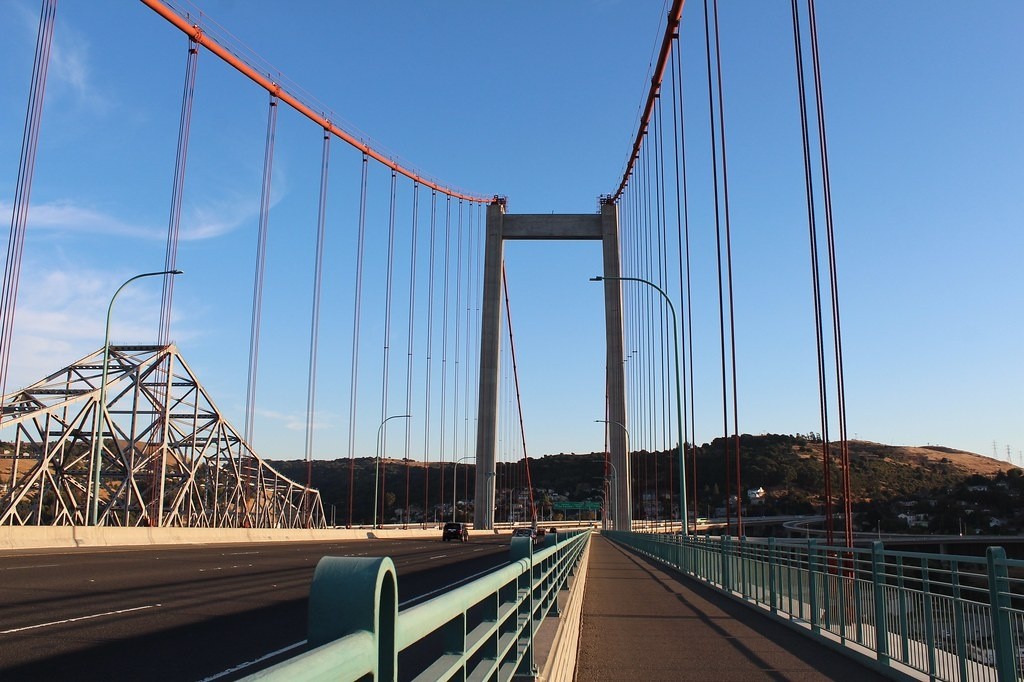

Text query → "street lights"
[92,269,186,526]
[374,415,414,529]
[588,275,689,534]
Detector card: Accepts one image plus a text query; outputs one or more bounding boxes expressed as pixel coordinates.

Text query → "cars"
[442,523,469,542]
[538,527,546,536]
[513,527,536,545]
